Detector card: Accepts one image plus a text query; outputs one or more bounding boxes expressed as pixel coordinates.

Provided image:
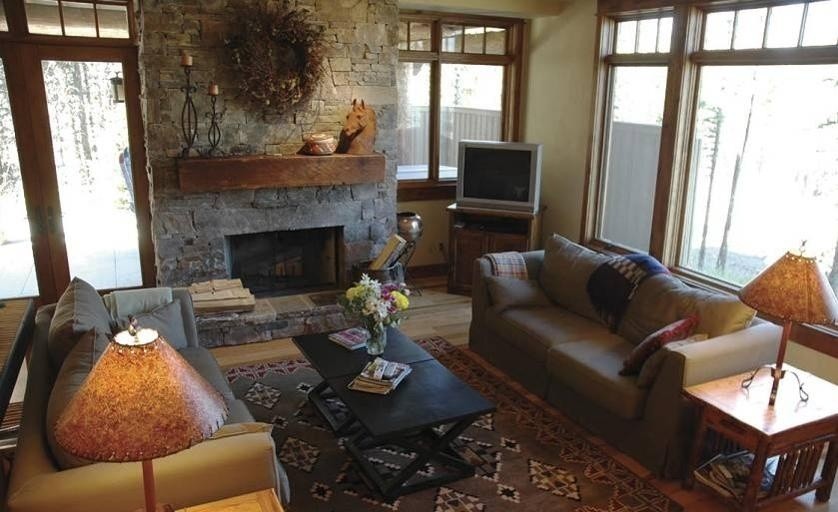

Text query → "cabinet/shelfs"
[444,201,549,297]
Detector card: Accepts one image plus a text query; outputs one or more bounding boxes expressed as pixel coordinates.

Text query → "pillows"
[483,273,553,316]
[45,277,113,374]
[635,332,710,390]
[616,308,701,379]
[115,297,187,350]
[44,324,112,471]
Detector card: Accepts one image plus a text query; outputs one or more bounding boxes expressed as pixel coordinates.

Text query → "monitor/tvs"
[456,139,543,214]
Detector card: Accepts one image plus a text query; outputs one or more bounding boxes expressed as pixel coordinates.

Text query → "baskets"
[310,133,338,155]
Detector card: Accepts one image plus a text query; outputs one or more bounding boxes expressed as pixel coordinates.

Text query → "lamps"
[736,238,838,407]
[109,70,126,104]
[53,314,231,512]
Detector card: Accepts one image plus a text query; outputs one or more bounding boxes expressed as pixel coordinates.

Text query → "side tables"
[174,487,286,511]
[678,359,838,511]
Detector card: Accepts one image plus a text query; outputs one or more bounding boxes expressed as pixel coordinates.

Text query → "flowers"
[343,270,414,351]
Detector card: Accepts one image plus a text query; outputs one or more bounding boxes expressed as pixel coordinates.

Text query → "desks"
[0,296,37,423]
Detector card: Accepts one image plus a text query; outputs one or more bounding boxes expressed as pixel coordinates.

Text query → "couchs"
[4,282,294,512]
[467,231,784,470]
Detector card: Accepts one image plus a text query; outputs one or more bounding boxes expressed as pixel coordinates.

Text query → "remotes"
[384,362,398,379]
[373,361,388,381]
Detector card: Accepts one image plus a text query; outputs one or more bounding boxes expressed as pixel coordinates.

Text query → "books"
[347,357,413,395]
[328,326,371,350]
[692,448,774,501]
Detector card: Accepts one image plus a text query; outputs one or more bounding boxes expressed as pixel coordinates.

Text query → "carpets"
[219,335,683,511]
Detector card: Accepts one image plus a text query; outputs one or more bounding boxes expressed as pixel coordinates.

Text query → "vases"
[362,320,388,356]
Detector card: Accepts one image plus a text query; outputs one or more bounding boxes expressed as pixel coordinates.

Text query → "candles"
[206,83,220,96]
[181,55,193,65]
